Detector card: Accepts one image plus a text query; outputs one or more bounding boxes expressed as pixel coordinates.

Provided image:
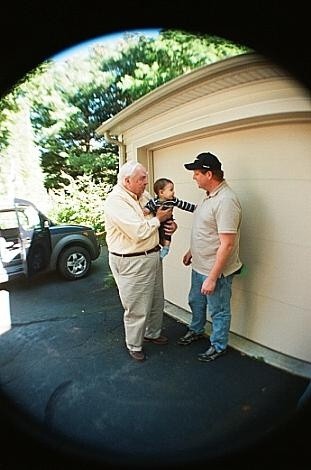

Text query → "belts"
[112,245,161,257]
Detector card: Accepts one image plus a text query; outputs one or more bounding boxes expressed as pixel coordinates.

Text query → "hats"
[184,152,221,170]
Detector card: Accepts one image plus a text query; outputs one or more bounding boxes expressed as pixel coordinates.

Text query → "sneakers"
[176,330,203,345]
[197,345,228,362]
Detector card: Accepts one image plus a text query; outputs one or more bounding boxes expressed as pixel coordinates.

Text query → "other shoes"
[130,350,144,359]
[143,335,169,344]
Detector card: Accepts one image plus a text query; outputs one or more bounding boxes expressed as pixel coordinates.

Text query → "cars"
[0,198,101,281]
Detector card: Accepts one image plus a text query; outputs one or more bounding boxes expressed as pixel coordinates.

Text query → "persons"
[104,162,178,361]
[176,153,243,361]
[143,177,197,257]
